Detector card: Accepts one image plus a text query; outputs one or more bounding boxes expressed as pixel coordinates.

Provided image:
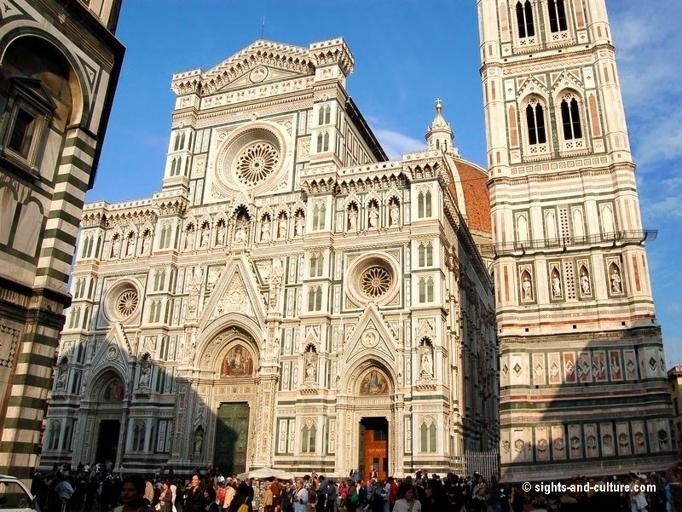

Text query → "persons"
[306,364,315,383]
[140,364,151,387]
[111,198,399,258]
[581,272,590,293]
[610,269,622,293]
[420,348,433,375]
[552,274,560,296]
[57,370,67,390]
[523,277,532,300]
[223,349,252,376]
[194,431,203,452]
